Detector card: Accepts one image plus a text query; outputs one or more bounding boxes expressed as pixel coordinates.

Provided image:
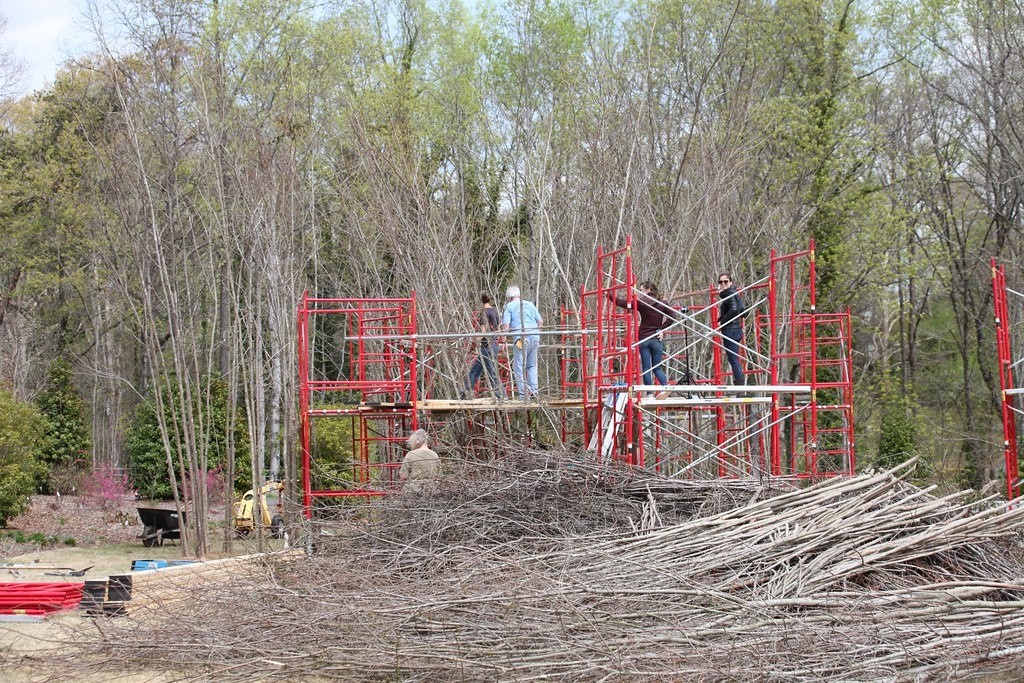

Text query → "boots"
[726,380,745,396]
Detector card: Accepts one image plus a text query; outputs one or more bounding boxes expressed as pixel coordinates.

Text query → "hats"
[505,286,520,299]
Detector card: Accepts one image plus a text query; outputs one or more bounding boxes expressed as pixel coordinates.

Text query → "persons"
[500,286,543,401]
[717,273,749,385]
[401,429,444,495]
[602,280,676,400]
[460,290,509,400]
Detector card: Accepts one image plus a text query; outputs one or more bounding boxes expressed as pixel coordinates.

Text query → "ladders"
[130,559,198,571]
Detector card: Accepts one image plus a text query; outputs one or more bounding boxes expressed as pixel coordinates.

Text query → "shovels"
[0,558,95,577]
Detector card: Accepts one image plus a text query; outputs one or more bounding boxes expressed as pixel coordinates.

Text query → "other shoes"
[656,391,671,401]
[455,389,465,400]
[645,393,654,399]
[492,394,508,402]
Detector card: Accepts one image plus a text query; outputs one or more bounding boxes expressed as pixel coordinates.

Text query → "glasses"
[718,280,730,284]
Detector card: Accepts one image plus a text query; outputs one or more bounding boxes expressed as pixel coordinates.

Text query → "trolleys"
[134,506,186,547]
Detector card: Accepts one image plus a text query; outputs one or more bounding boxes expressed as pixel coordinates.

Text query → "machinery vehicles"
[232,478,300,540]
